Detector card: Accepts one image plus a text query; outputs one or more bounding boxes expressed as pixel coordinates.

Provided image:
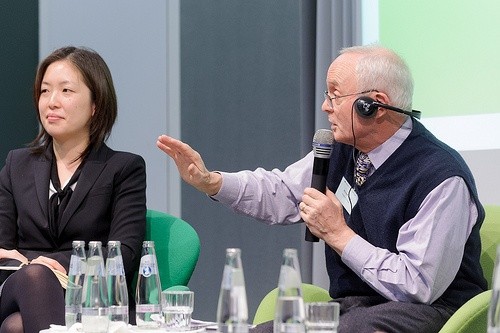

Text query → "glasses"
[323,90,379,109]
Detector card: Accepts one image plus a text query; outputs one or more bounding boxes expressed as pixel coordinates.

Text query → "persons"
[156,45,488,333]
[0,46,147,333]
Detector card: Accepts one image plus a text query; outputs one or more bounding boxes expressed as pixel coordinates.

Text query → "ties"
[353,152,373,187]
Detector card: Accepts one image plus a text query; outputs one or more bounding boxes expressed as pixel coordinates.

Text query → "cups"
[160,291,195,333]
[305,300,340,333]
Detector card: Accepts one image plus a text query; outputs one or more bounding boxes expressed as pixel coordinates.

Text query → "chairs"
[252,202,500,333]
[131,210,201,290]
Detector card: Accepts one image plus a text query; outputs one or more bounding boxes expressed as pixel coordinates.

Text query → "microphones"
[304,129,334,242]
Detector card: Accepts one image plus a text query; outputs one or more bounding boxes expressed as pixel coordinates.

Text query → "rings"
[301,205,306,212]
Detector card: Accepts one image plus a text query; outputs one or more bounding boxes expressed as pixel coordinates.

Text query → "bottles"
[134,241,163,331]
[80,241,109,319]
[64,240,87,329]
[105,241,130,326]
[272,248,306,333]
[216,248,250,333]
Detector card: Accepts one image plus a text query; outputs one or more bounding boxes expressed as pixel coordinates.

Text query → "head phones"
[355,98,422,119]
